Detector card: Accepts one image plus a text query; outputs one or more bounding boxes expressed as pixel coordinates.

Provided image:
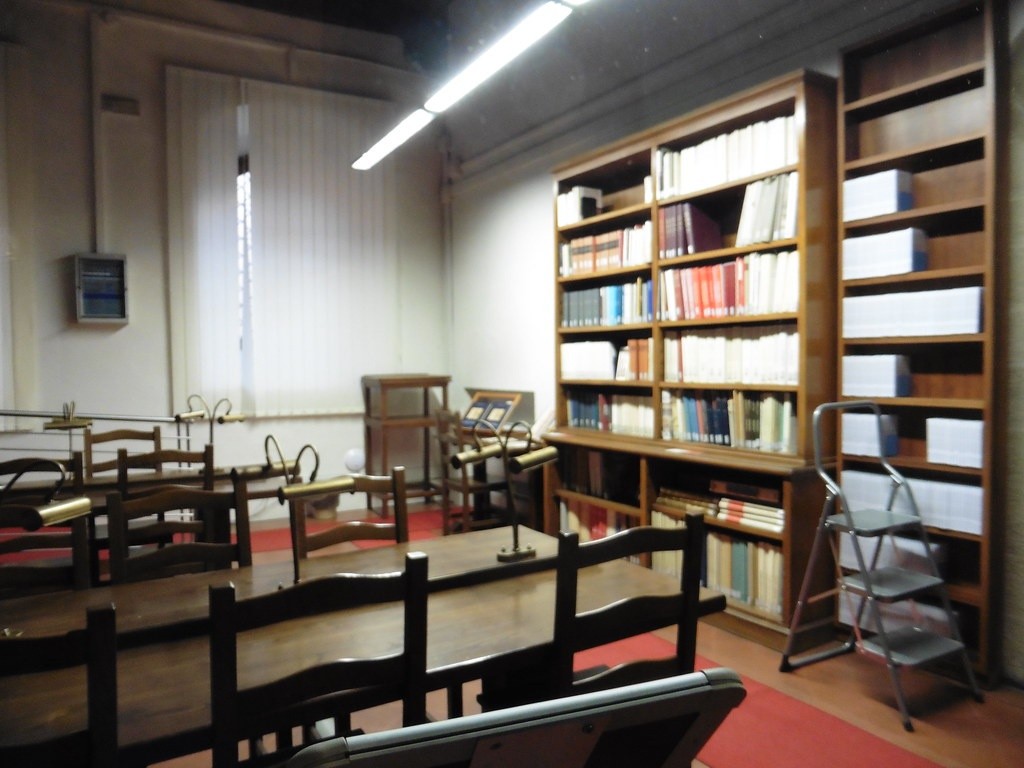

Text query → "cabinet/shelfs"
[544,1,1017,661]
[361,374,451,521]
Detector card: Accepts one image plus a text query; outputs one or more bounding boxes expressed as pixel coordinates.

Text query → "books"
[555,114,799,456]
[838,167,985,639]
[558,442,786,620]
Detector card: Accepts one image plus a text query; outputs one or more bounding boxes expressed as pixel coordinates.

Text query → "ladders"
[779,399,988,734]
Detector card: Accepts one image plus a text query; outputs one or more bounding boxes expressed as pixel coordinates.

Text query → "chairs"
[0,411,750,768]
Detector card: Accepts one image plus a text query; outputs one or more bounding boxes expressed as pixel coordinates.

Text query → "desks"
[0,521,727,768]
